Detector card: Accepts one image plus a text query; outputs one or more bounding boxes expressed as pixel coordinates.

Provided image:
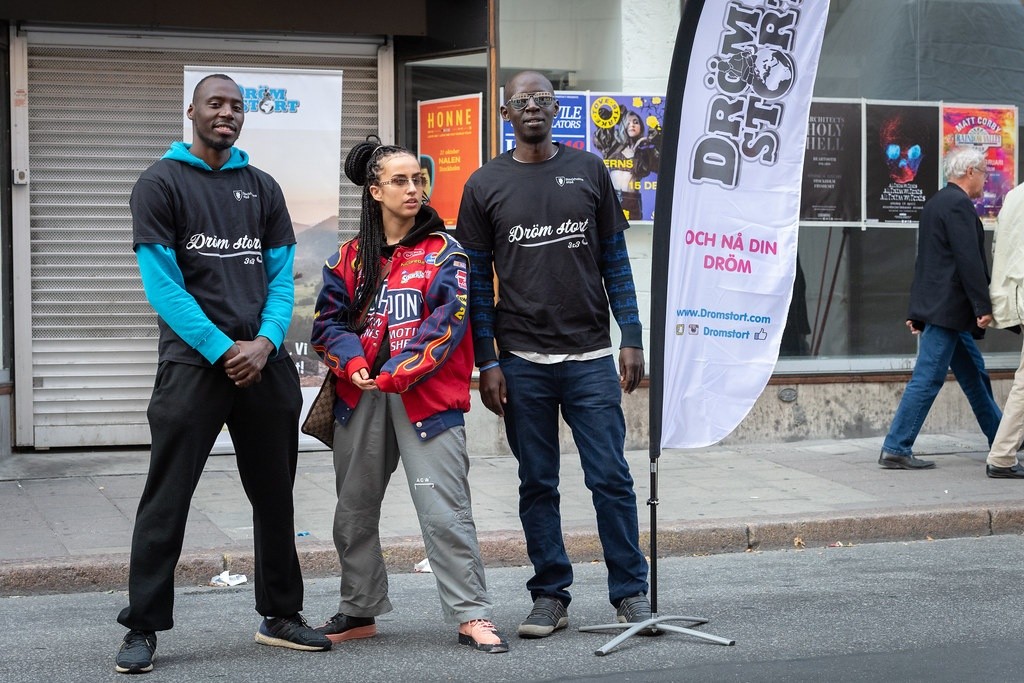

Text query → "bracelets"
[479,362,501,373]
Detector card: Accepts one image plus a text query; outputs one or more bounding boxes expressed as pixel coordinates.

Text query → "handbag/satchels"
[302,367,337,452]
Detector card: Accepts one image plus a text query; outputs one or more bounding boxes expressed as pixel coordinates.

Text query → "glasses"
[379,177,422,186]
[506,92,555,110]
[975,167,990,179]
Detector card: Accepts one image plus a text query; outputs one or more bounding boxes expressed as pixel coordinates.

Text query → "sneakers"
[518,595,569,636]
[617,592,665,634]
[459,619,508,652]
[313,612,377,642]
[116,629,157,672]
[255,612,332,650]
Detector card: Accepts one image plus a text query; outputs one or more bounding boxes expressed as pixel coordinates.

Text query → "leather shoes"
[1017,441,1024,451]
[987,463,1024,479]
[879,451,936,469]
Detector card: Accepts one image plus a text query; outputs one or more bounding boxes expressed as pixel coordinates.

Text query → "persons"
[456,71,665,636]
[310,134,509,652]
[593,106,660,222]
[986,185,1024,478]
[115,74,332,674]
[878,147,1024,469]
